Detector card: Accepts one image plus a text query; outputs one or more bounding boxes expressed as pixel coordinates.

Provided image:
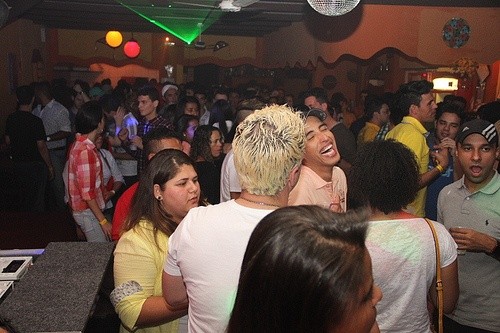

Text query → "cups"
[449,225,467,255]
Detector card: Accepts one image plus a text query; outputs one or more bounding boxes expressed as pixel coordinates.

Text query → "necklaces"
[239,196,280,208]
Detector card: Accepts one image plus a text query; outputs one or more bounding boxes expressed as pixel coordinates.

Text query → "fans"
[173,0,259,12]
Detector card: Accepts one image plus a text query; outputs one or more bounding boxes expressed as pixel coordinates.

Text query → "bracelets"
[47,136,51,142]
[99,219,107,225]
[110,190,115,194]
[436,164,443,172]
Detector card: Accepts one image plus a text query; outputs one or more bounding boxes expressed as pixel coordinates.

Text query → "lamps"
[165,22,229,51]
[106,30,123,48]
[123,31,142,58]
[307,0,361,17]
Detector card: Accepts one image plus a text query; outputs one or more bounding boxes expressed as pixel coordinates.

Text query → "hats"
[455,119,498,150]
[162,85,178,97]
[300,108,327,123]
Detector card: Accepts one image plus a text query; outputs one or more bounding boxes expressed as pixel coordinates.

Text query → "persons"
[0,79,500,333]
